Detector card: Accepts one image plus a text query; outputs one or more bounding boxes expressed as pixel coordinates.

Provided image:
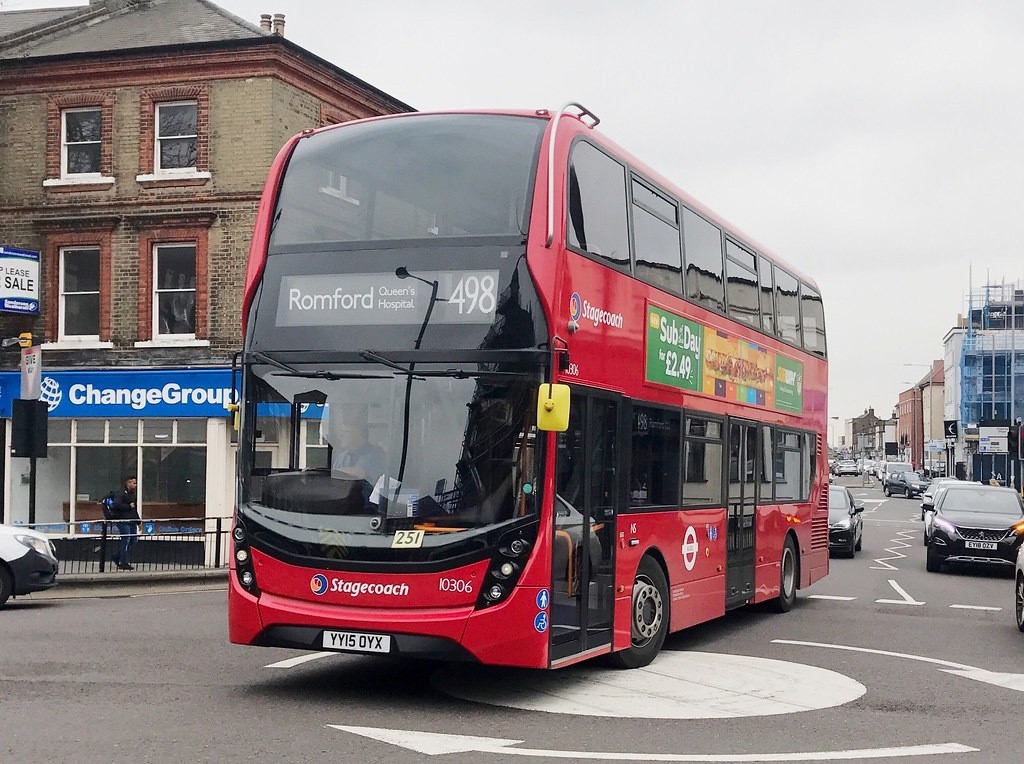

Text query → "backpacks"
[102,490,129,520]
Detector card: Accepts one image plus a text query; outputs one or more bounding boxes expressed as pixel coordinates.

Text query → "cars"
[923,484,1024,574]
[828,485,864,559]
[0,523,60,607]
[920,477,985,547]
[882,470,927,499]
[1014,542,1024,633]
[828,458,913,485]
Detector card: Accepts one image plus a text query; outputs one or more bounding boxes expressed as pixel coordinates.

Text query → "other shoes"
[113,554,120,563]
[118,563,134,570]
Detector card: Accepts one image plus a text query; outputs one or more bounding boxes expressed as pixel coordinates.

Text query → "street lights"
[904,363,932,479]
[901,380,918,470]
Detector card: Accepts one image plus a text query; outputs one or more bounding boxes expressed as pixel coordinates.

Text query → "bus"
[228,100,831,673]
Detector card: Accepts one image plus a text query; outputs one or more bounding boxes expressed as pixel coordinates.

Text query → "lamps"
[2,338,34,347]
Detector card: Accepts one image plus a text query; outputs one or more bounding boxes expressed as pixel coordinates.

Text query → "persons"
[111,476,142,570]
[331,416,388,487]
[911,459,916,472]
[989,479,1001,486]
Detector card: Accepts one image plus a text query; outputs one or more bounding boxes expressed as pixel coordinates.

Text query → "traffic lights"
[1007,424,1020,461]
